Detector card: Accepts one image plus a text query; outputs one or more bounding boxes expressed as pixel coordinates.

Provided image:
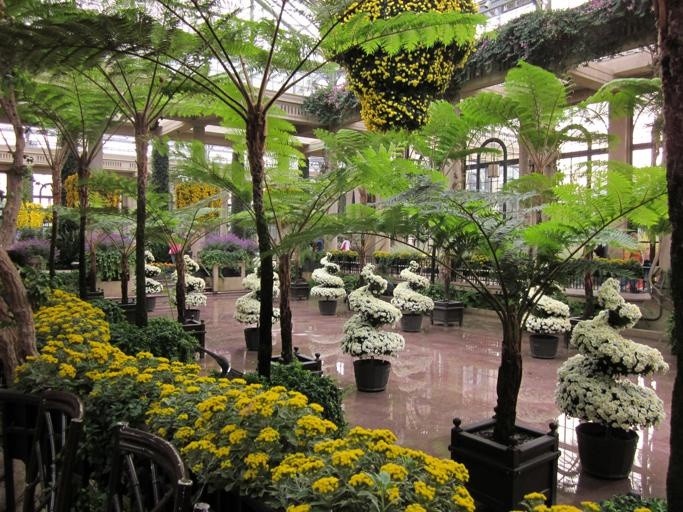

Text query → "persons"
[314,233,326,254]
[340,235,350,251]
[593,230,611,286]
[626,232,644,290]
